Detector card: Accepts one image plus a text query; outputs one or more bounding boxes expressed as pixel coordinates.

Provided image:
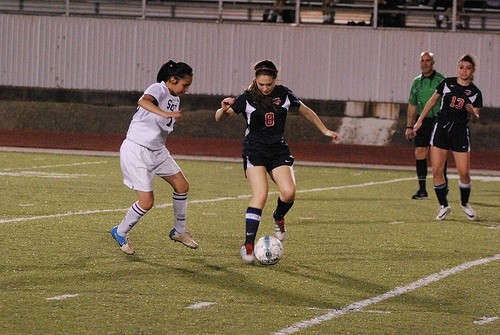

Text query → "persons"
[215,59,340,264]
[111,60,199,255]
[405,51,449,200]
[413,53,483,222]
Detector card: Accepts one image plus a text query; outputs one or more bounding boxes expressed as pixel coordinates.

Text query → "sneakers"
[436,203,451,221]
[462,203,475,219]
[112,225,135,255]
[412,189,428,200]
[239,245,254,263]
[170,228,197,248]
[273,218,285,241]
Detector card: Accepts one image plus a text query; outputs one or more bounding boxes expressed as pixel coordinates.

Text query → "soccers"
[254,235,284,265]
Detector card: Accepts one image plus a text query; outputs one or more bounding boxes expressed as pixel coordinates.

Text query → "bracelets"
[406,126,414,129]
